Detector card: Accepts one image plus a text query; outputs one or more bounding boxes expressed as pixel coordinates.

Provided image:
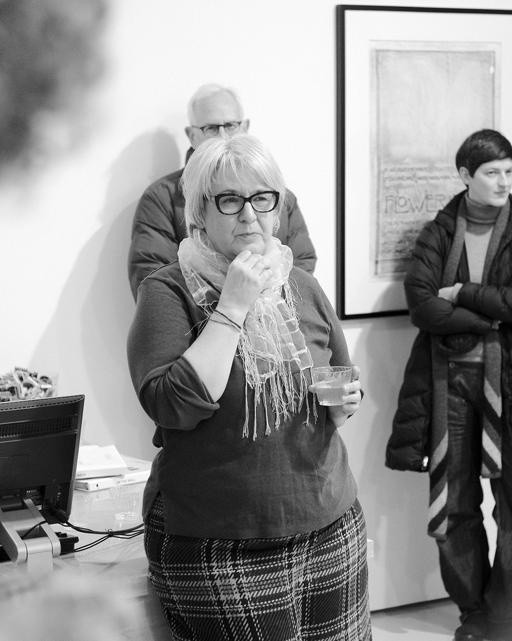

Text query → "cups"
[109,486,146,523]
[312,364,354,408]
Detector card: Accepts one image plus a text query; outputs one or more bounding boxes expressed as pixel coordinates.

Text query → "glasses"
[203,190,279,215]
[192,121,242,138]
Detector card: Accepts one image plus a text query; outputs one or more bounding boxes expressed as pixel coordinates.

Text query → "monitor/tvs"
[0,394,88,569]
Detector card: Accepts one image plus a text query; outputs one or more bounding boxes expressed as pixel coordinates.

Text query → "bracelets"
[209,310,244,332]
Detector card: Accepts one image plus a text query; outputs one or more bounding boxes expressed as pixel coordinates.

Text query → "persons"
[126,133,372,641]
[128,83,318,303]
[385,130,512,641]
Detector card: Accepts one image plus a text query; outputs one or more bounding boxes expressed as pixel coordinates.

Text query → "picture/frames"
[334,4,512,322]
